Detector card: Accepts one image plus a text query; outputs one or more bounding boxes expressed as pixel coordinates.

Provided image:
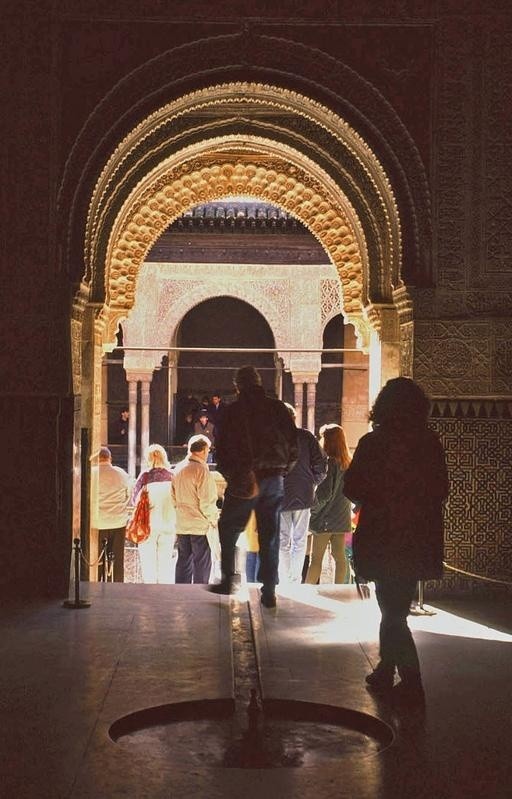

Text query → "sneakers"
[364,668,396,690]
[207,580,234,596]
[382,680,424,706]
[260,591,277,608]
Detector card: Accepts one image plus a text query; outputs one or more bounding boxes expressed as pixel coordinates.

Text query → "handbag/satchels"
[122,473,152,545]
[225,420,259,501]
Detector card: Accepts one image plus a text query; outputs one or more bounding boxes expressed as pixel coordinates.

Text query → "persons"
[335,374,449,699]
[90,386,365,583]
[203,365,302,608]
